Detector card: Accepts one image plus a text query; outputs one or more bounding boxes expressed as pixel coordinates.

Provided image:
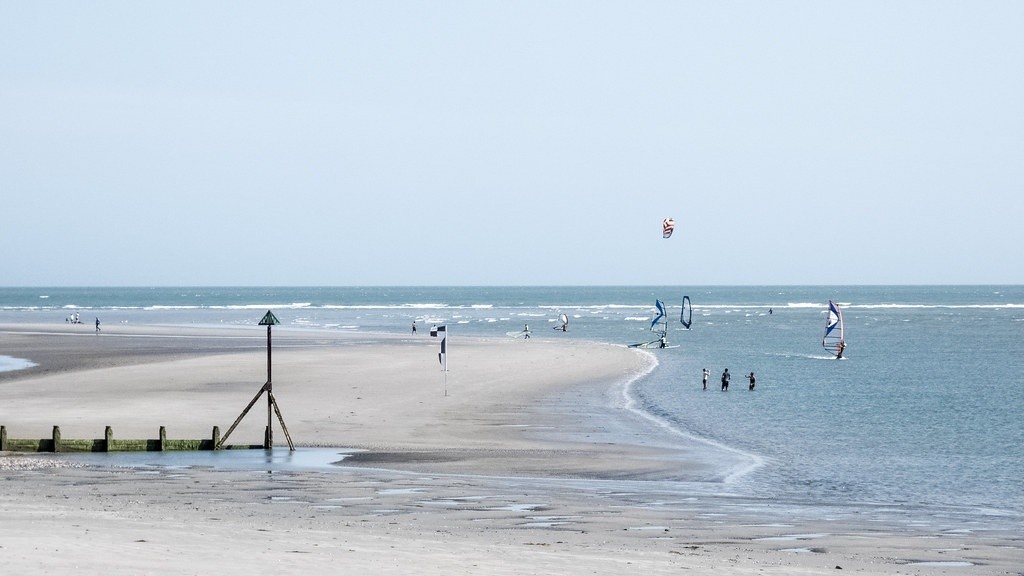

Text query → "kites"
[661,217,675,239]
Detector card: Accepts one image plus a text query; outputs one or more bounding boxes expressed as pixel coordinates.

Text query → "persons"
[721,368,731,391]
[561,323,566,331]
[95,317,101,331]
[838,341,847,358]
[745,371,755,391]
[412,321,417,335]
[769,308,773,314]
[659,334,666,348]
[703,368,711,390]
[524,323,531,339]
[65,311,83,324]
